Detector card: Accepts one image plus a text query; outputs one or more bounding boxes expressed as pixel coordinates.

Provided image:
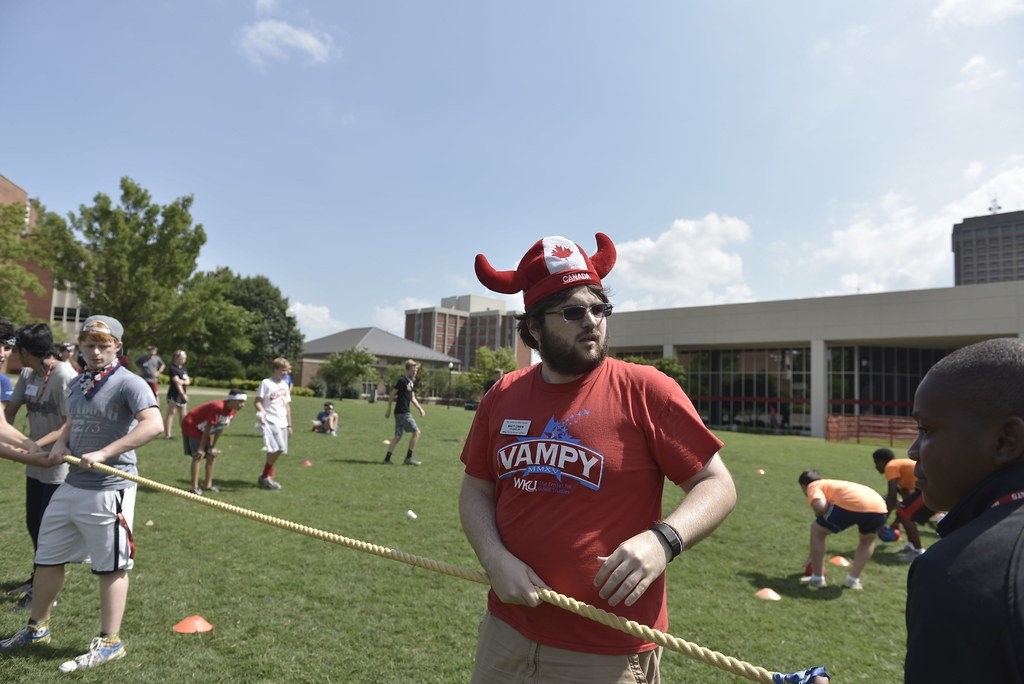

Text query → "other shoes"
[258,475,281,490]
[190,486,219,495]
[163,436,174,440]
[404,458,421,466]
[326,429,337,437]
[6,572,58,612]
[382,459,395,464]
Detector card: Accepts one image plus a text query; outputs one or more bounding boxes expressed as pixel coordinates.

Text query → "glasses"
[541,304,614,322]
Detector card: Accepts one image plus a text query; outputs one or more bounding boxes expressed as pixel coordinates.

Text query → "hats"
[81,315,124,357]
[475,232,616,312]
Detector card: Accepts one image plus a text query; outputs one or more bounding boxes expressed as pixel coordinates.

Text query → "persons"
[481,368,503,398]
[312,402,339,436]
[468,232,739,684]
[798,470,889,590]
[384,358,425,465]
[0,313,294,673]
[903,337,1024,684]
[872,448,946,563]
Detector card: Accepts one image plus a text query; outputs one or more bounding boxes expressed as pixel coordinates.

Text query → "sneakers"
[844,574,862,590]
[799,574,827,589]
[893,541,925,561]
[0,619,51,650]
[58,636,127,674]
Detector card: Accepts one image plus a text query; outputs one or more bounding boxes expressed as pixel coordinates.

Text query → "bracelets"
[650,520,682,562]
[287,426,293,428]
